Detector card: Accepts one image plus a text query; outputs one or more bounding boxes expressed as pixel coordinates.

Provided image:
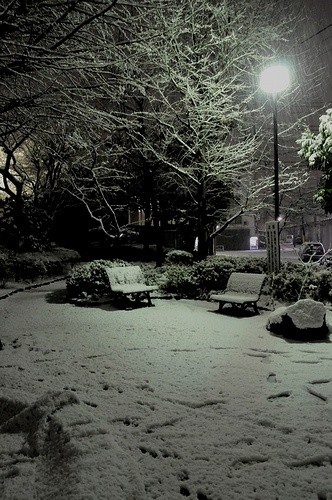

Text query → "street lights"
[258,62,291,221]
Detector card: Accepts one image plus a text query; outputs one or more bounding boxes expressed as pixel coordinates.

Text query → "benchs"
[209,271,267,314]
[105,266,160,309]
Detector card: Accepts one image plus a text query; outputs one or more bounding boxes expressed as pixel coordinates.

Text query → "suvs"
[298,242,325,263]
